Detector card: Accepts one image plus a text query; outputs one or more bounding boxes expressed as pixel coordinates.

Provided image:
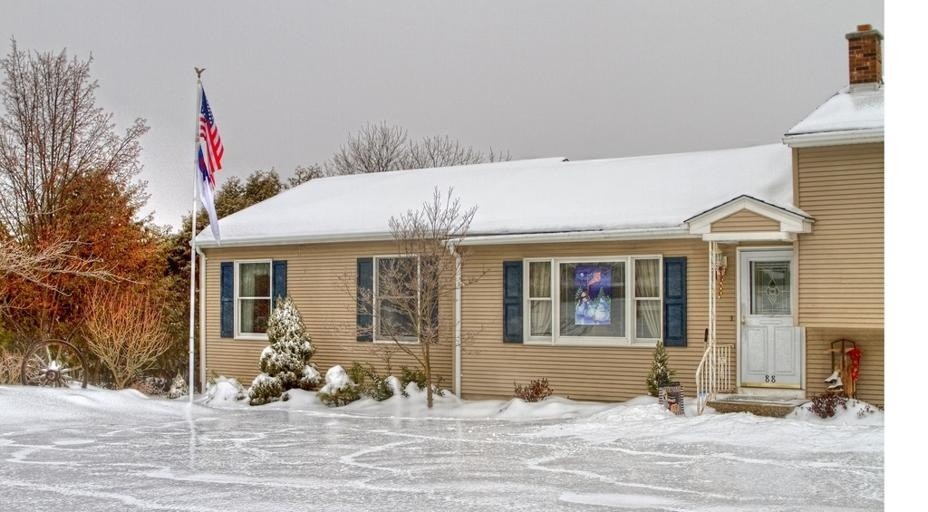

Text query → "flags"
[196,84,224,245]
